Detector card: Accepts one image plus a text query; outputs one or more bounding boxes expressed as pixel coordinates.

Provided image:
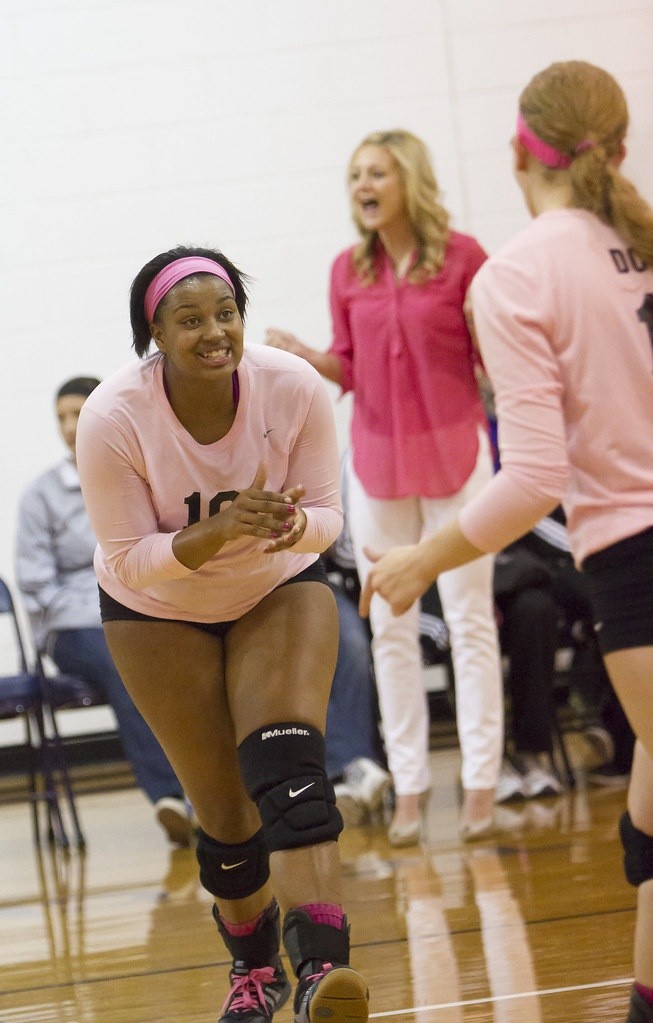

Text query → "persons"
[267,64,653,1023]
[15,377,199,847]
[76,247,374,1023]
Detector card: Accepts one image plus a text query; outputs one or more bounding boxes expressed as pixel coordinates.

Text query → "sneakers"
[217,967,291,1023]
[520,752,561,799]
[294,962,370,1023]
[494,757,523,801]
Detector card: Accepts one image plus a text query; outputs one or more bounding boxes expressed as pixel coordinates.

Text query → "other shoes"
[333,784,362,824]
[347,758,392,811]
[562,726,614,770]
[156,796,191,841]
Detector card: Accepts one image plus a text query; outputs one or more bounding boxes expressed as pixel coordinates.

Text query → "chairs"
[0,579,602,849]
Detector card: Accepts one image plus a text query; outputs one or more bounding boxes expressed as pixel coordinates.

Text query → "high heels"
[460,815,490,838]
[388,793,429,844]
